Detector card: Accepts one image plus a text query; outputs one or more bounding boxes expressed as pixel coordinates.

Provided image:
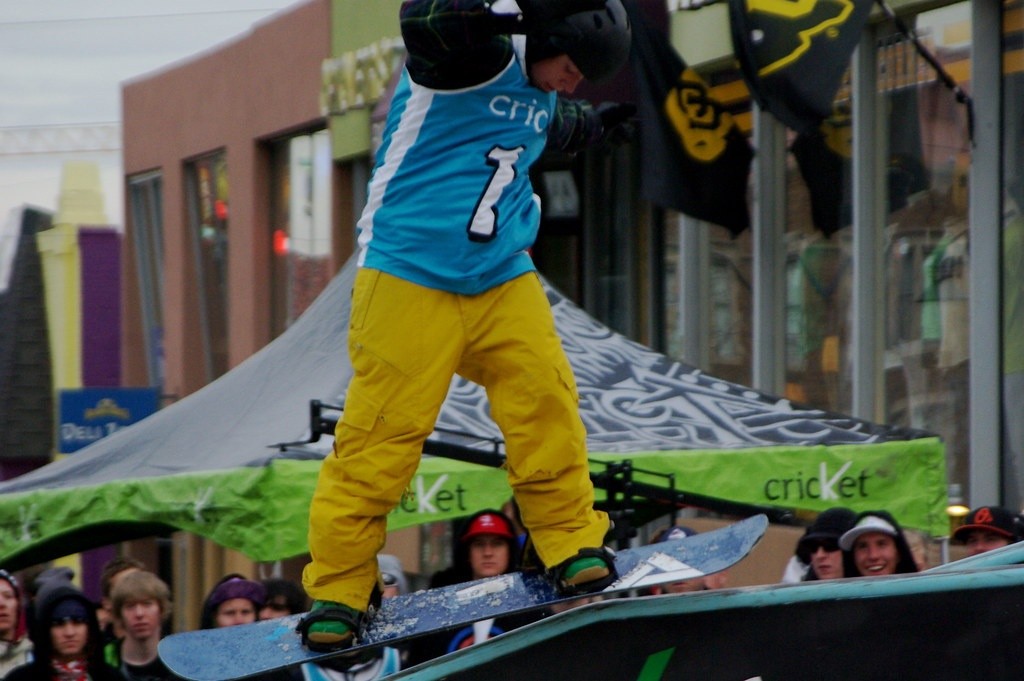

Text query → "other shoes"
[305,600,361,643]
[564,557,609,586]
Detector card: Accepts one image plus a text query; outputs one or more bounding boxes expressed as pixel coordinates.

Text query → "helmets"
[544,0,633,85]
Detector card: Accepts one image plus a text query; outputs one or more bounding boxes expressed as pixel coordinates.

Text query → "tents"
[0,247,951,566]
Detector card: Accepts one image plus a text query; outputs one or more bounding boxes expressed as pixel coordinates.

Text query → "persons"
[951,505,1024,557]
[646,523,712,595]
[0,495,608,681]
[793,504,919,581]
[296,0,639,654]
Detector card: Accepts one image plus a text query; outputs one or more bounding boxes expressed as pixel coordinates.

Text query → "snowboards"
[154,510,771,681]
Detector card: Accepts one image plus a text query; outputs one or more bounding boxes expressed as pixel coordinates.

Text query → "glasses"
[52,616,84,626]
[805,542,841,553]
[379,573,398,585]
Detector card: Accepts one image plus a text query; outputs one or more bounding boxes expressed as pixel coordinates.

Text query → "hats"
[838,515,899,551]
[462,514,513,541]
[801,508,857,539]
[207,577,266,608]
[952,506,1015,545]
[51,598,88,616]
